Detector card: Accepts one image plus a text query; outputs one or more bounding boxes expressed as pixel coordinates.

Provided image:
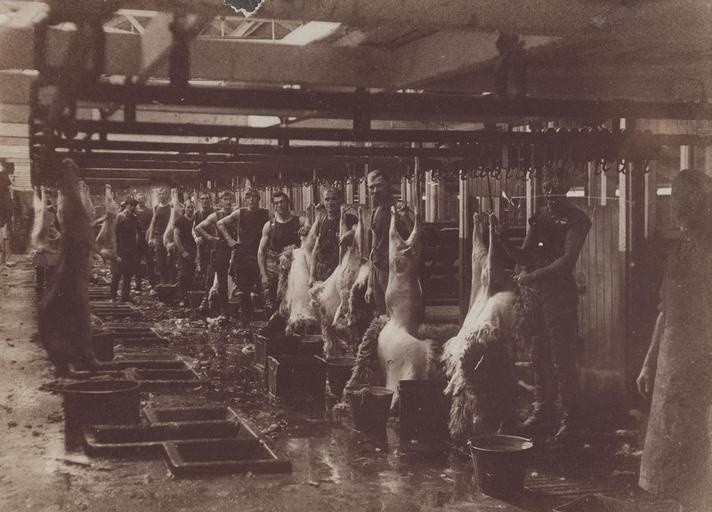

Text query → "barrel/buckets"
[467,433,534,502]
[345,384,394,433]
[326,353,360,393]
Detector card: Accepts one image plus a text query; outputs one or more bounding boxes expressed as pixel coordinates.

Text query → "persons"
[635,168,712,511]
[515,177,591,439]
